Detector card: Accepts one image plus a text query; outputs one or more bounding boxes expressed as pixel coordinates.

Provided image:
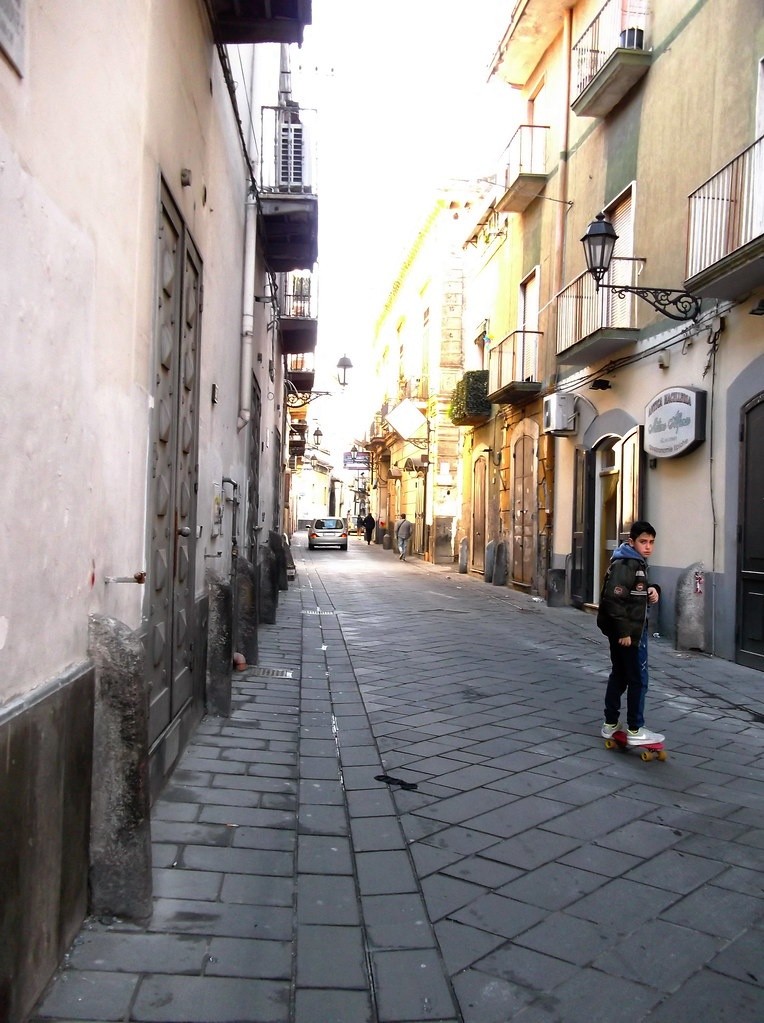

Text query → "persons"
[357,515,363,536]
[394,514,412,561]
[347,510,350,518]
[600,521,666,746]
[363,513,375,545]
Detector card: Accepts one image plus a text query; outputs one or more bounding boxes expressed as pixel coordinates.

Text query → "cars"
[348,516,365,535]
[306,517,348,551]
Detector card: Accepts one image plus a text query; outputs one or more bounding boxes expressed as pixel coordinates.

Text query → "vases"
[577,76,593,92]
[618,28,644,48]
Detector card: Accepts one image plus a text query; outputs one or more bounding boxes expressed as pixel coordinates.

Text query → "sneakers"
[601,721,623,738]
[627,726,665,745]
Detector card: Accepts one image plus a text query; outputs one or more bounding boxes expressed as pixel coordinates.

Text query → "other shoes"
[399,553,403,559]
[402,558,406,560]
[368,542,370,544]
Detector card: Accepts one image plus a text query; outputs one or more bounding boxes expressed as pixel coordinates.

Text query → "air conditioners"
[274,120,308,190]
[542,392,574,431]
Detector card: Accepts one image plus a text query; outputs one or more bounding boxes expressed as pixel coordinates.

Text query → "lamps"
[580,211,698,323]
[305,427,323,448]
[302,455,317,471]
[351,445,373,464]
[589,379,611,391]
[285,354,354,408]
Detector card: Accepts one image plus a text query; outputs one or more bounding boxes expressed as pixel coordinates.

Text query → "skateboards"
[604,730,667,761]
